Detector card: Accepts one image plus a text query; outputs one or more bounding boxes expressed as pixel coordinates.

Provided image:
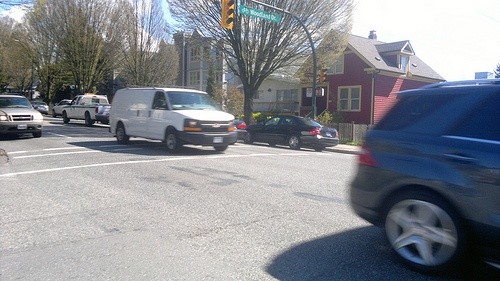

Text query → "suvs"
[348,78,500,274]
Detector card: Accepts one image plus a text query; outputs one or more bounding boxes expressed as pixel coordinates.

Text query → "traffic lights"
[221,0,236,30]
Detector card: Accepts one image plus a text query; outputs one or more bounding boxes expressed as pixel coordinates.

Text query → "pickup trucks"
[61,94,109,127]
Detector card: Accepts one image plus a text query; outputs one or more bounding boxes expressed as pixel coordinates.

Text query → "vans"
[109,86,238,153]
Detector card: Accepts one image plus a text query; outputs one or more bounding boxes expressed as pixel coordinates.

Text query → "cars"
[52,99,72,118]
[0,95,43,138]
[31,101,49,115]
[234,120,247,137]
[242,114,340,153]
